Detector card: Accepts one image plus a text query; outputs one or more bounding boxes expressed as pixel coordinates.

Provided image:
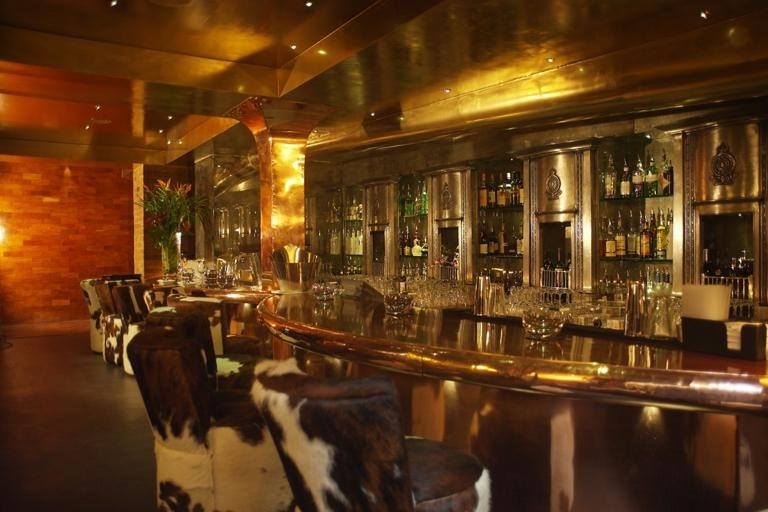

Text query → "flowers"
[132,178,210,279]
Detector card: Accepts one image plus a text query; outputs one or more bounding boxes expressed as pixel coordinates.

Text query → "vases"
[162,232,182,280]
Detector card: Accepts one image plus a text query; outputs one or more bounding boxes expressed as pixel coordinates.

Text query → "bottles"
[399,179,428,283]
[703,249,753,297]
[321,189,365,275]
[555,247,564,287]
[479,170,524,287]
[602,149,672,300]
[398,269,407,295]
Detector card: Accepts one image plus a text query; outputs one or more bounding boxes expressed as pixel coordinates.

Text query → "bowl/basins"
[521,311,564,340]
[384,294,414,316]
[312,283,337,301]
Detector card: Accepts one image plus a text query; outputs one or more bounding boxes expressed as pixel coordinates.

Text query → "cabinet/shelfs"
[310,97,767,321]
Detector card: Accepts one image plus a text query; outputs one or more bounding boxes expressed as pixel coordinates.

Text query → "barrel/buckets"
[269,244,322,294]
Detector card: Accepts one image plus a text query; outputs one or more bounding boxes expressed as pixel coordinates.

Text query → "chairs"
[126,321,299,510]
[78,275,261,382]
[150,308,272,399]
[249,356,491,511]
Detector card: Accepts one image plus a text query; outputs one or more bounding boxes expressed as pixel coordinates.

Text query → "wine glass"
[364,275,466,310]
[507,286,595,313]
[198,259,208,291]
[177,253,186,284]
[217,264,227,292]
